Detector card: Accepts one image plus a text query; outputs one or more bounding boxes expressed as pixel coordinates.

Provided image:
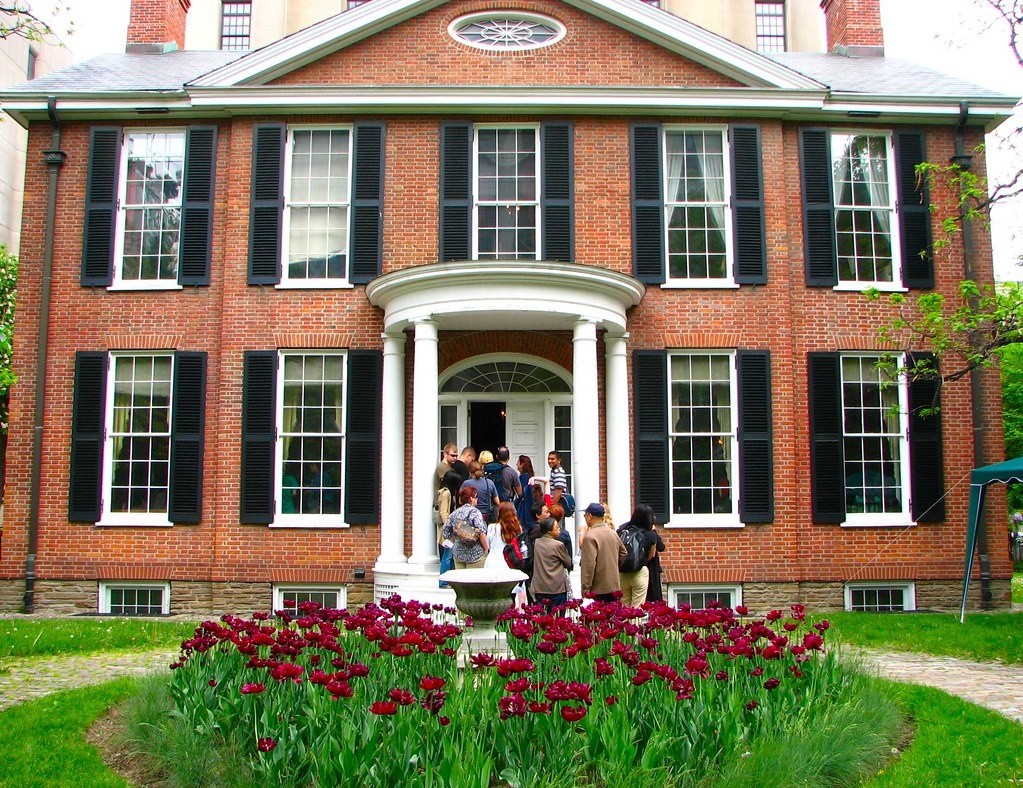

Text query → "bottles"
[521,540,530,559]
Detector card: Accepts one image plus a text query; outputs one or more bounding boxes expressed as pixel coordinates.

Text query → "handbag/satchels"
[559,494,576,518]
[432,506,442,524]
[486,507,499,523]
[453,518,481,545]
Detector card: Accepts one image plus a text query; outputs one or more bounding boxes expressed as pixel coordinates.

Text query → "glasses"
[472,495,478,499]
[445,451,458,457]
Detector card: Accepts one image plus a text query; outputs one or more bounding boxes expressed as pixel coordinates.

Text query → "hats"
[584,503,605,517]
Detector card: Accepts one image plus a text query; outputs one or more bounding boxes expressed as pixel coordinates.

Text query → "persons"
[305,463,333,513]
[432,443,574,623]
[579,502,628,604]
[714,463,729,506]
[282,467,299,513]
[616,504,665,610]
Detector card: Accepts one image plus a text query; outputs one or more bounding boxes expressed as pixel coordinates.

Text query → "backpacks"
[503,524,536,569]
[484,461,510,502]
[618,522,647,573]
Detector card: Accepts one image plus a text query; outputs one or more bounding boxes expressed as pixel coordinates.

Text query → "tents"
[960,457,1023,624]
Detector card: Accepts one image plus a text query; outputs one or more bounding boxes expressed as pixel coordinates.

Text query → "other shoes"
[440,585,452,589]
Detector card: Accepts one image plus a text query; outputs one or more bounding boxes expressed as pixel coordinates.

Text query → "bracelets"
[579,531,583,535]
